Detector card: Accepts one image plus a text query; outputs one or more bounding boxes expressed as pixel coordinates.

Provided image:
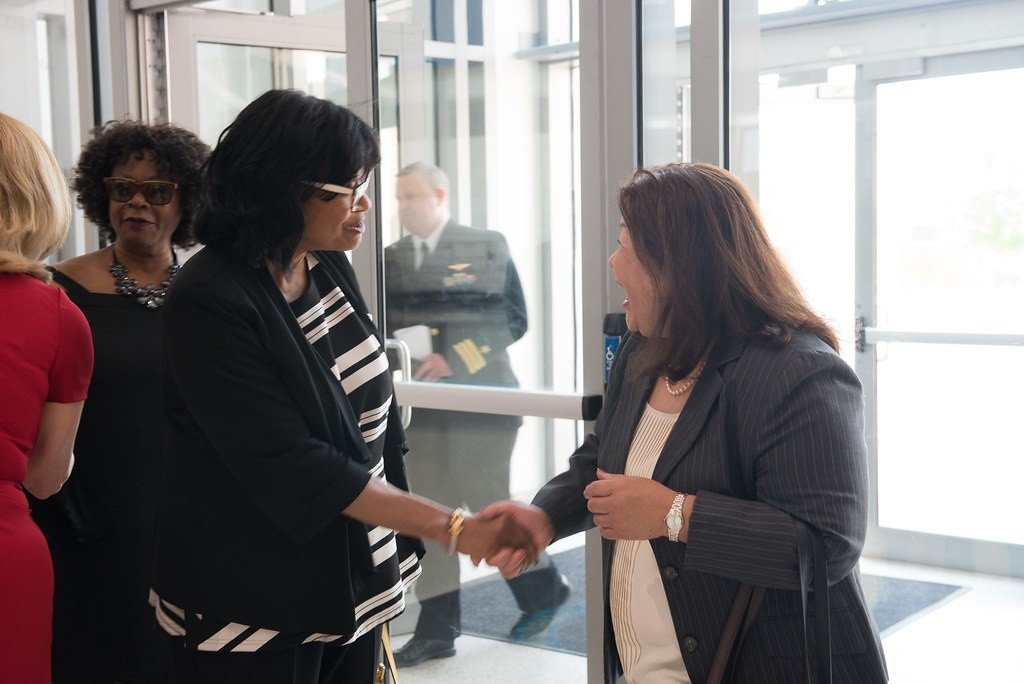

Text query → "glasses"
[103,176,179,205]
[300,172,371,209]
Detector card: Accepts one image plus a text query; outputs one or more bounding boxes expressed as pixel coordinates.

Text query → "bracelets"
[447,507,467,556]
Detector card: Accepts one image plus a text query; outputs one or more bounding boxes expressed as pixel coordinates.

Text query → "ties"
[419,243,429,275]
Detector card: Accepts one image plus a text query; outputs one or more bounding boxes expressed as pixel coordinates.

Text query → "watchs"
[663,492,689,543]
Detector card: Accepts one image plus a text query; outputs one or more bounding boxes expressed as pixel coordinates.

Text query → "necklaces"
[110,248,180,311]
[664,360,705,395]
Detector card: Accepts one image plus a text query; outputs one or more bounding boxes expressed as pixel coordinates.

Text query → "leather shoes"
[392,635,456,666]
[512,575,571,639]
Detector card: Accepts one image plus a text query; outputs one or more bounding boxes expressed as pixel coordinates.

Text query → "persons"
[40,116,211,683]
[470,163,890,684]
[385,161,570,668]
[137,87,538,684]
[0,112,93,683]
